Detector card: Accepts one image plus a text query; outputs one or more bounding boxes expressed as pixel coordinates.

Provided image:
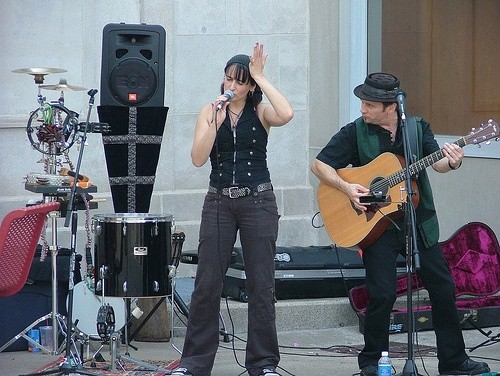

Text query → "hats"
[353,72,406,102]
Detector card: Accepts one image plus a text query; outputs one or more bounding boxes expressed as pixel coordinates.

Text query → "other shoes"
[360,365,378,376]
[438,356,490,375]
[259,368,282,376]
[171,367,193,376]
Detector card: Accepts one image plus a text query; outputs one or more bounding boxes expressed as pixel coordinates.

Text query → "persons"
[310,72,491,376]
[171,42,293,376]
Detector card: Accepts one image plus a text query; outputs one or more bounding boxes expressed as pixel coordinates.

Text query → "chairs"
[0,200,63,298]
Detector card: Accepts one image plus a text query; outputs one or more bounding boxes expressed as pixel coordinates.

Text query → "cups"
[39,326,53,353]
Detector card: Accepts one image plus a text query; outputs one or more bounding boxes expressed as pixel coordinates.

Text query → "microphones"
[216,90,234,111]
[396,90,407,123]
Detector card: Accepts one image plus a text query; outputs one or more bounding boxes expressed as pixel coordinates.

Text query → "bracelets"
[448,159,462,170]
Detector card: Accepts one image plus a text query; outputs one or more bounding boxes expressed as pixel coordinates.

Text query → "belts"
[208,183,273,199]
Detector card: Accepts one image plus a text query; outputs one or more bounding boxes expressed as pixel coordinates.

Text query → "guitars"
[317,118,500,251]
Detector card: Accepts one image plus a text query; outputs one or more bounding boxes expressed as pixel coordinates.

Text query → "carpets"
[322,340,438,360]
[31,354,181,376]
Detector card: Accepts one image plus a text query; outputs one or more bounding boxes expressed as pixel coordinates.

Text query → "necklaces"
[227,106,245,129]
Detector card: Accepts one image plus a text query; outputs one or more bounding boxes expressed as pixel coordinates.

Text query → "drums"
[90,213,175,299]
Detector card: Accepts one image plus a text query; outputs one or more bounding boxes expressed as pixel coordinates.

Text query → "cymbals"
[97,303,115,342]
[23,173,74,187]
[11,67,70,76]
[37,84,87,92]
[27,104,79,155]
[77,122,112,134]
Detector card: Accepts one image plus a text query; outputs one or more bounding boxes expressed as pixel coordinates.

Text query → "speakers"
[100,23,166,107]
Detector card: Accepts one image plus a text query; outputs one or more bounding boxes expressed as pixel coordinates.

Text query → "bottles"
[378,351,392,376]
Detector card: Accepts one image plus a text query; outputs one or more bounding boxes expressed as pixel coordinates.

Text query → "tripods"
[0,78,101,376]
